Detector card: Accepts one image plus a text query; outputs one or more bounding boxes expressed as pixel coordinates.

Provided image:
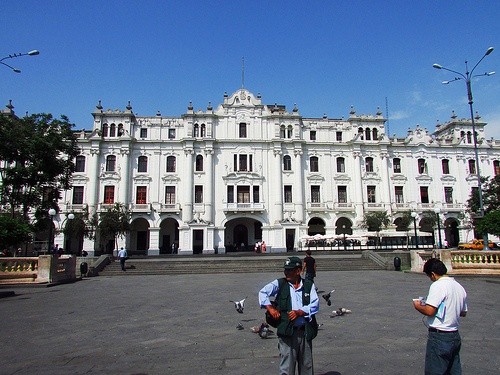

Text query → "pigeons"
[229,295,248,314]
[329,307,351,318]
[237,322,243,329]
[250,322,274,338]
[322,292,331,306]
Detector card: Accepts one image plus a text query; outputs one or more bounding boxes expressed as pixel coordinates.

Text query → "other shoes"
[316,287,318,294]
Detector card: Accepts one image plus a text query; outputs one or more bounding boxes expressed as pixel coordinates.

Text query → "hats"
[280,256,302,268]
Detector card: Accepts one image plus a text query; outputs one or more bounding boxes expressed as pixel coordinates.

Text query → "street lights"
[433,208,443,249]
[410,211,418,250]
[432,46,496,250]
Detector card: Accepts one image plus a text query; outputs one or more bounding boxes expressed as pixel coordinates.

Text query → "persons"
[81,250,88,257]
[254,241,267,254]
[258,256,319,375]
[171,241,177,255]
[443,239,450,249]
[118,247,127,271]
[412,258,468,375]
[301,250,318,293]
[53,244,59,257]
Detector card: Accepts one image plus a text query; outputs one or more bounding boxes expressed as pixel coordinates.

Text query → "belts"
[428,327,458,333]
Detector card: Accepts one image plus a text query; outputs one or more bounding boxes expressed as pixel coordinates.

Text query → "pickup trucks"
[457,239,494,250]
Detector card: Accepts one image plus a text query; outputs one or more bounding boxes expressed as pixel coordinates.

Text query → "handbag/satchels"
[265,301,279,328]
